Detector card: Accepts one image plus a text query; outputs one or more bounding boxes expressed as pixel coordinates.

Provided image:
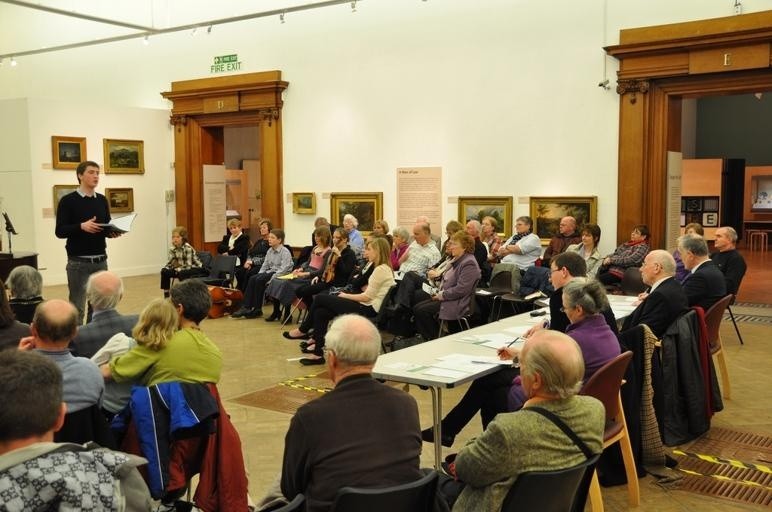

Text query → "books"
[96,212,138,234]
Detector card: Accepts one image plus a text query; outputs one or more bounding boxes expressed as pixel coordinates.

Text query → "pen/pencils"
[643,287,649,296]
[497,336,520,356]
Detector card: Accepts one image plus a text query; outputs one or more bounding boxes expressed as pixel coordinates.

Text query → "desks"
[0,251,39,284]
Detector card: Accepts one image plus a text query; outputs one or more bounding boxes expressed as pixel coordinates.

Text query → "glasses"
[642,262,658,268]
[546,268,562,279]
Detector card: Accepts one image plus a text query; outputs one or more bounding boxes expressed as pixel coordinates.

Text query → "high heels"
[281,314,293,325]
[265,310,283,322]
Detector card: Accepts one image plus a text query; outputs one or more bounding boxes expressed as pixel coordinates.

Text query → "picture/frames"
[103,138,145,174]
[457,196,512,241]
[292,192,316,214]
[53,184,79,215]
[51,135,87,170]
[104,187,134,213]
[329,192,383,236]
[529,196,598,246]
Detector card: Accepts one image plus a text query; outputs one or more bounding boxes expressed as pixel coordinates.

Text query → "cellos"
[206,284,244,319]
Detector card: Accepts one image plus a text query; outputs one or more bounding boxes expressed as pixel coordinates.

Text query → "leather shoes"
[441,452,458,477]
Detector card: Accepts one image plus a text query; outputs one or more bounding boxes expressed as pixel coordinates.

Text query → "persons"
[543,216,605,283]
[418,276,620,447]
[381,217,500,348]
[598,223,746,363]
[498,216,544,272]
[0,257,221,511]
[420,330,606,510]
[278,311,422,510]
[263,213,441,365]
[217,212,294,319]
[160,226,205,299]
[55,162,126,326]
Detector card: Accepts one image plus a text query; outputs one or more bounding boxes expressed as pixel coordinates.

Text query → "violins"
[321,238,342,283]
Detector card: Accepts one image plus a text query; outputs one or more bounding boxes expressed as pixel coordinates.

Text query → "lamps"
[0,0,358,67]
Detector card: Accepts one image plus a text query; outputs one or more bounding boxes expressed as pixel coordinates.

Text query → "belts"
[68,254,107,264]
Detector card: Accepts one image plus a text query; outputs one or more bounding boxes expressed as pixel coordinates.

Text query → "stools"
[751,232,768,254]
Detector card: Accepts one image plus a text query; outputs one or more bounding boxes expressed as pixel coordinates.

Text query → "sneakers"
[243,308,263,318]
[231,307,253,318]
[421,422,457,448]
[282,330,310,340]
[298,356,326,366]
[299,341,316,353]
